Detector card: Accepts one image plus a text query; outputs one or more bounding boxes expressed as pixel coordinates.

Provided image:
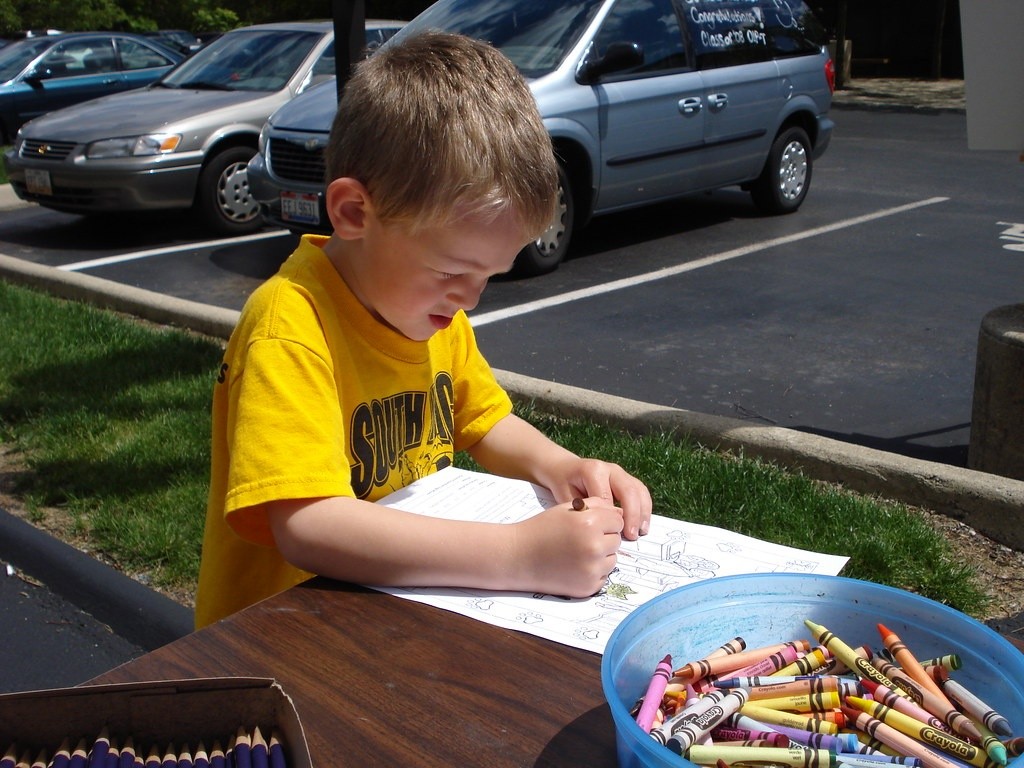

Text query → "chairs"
[610,11,672,71]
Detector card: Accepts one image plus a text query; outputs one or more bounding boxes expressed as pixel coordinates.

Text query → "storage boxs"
[0,677,313,768]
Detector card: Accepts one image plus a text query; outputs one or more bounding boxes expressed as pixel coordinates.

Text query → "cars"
[1,18,451,238]
[0,29,227,145]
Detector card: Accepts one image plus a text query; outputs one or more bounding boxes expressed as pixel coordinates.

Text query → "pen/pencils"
[0,720,289,768]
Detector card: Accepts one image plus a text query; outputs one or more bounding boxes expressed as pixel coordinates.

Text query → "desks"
[0,490,738,768]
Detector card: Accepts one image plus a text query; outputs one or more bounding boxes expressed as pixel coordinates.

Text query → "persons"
[193,29,654,639]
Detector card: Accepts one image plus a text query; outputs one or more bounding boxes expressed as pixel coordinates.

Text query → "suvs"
[246,0,838,275]
